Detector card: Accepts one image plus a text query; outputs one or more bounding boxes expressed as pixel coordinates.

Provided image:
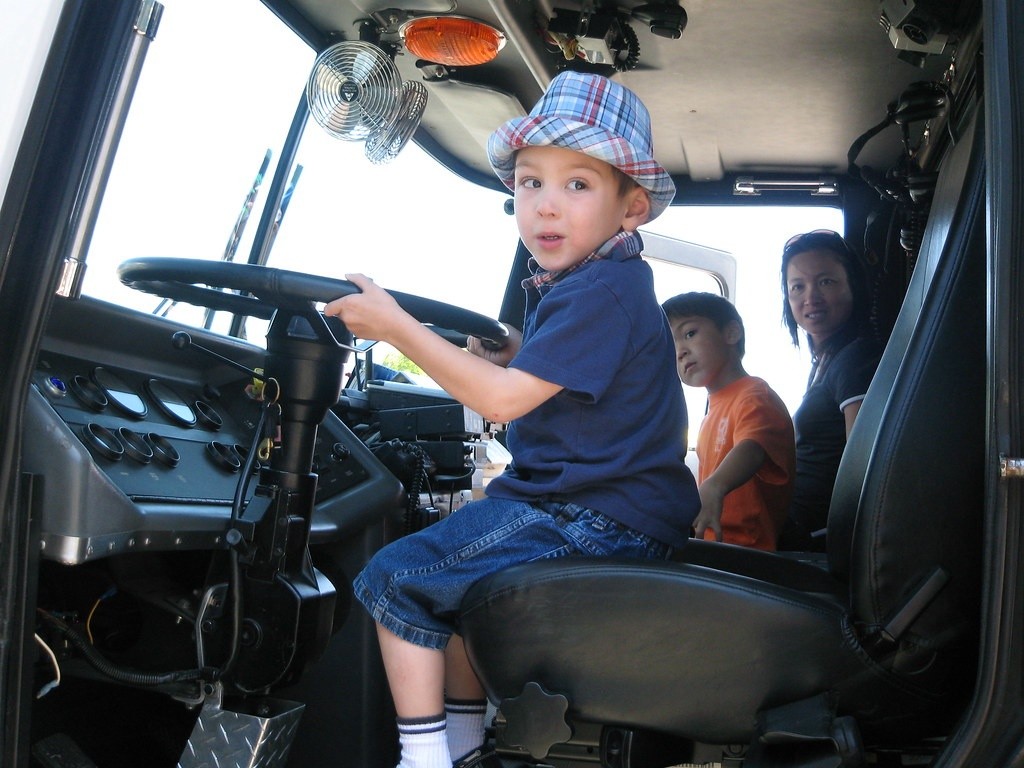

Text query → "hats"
[488,71,676,225]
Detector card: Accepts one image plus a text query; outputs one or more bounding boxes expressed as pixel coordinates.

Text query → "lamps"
[400,13,508,68]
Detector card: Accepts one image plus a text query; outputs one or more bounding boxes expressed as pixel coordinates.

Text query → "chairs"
[443,81,987,768]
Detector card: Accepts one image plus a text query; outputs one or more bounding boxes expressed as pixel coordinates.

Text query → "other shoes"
[452,745,496,768]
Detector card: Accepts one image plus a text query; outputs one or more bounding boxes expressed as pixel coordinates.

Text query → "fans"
[304,18,405,143]
[363,38,429,166]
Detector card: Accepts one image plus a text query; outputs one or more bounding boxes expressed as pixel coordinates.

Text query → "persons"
[779,228,878,549]
[658,289,803,556]
[324,71,703,768]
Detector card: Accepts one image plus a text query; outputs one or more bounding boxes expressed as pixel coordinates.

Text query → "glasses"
[783,229,850,255]
